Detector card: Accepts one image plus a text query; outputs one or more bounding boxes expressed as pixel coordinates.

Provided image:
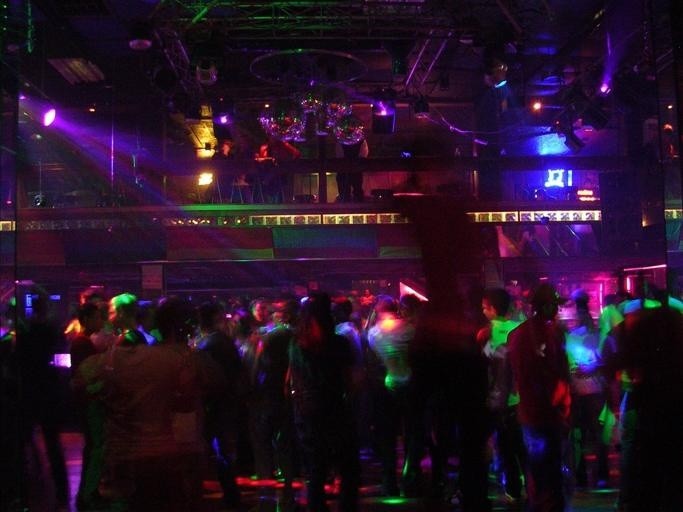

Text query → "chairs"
[190,144,290,204]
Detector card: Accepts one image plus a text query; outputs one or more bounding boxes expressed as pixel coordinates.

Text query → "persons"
[1,279,682,510]
[259,144,267,158]
[331,118,369,203]
[263,132,301,201]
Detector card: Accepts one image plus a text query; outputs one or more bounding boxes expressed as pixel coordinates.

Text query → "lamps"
[439,65,450,90]
[413,94,430,118]
